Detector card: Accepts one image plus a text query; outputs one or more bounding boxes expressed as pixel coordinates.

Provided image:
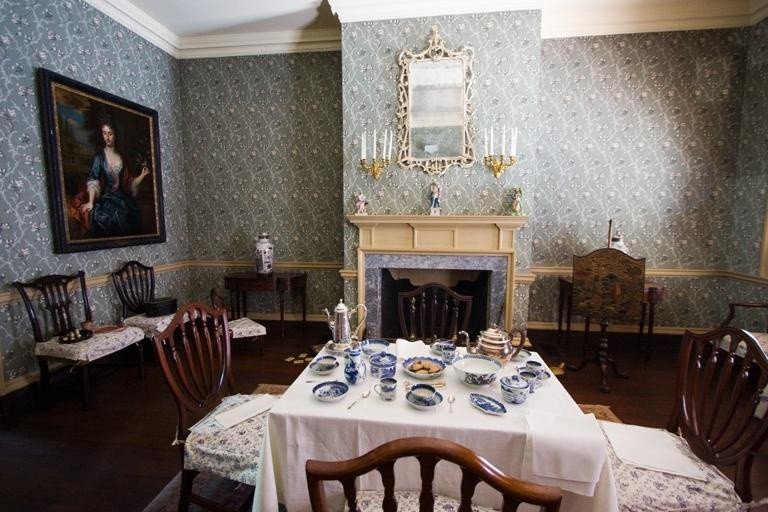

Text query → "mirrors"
[386,22,478,179]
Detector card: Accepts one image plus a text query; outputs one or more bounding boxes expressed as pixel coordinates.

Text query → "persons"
[80,117,150,237]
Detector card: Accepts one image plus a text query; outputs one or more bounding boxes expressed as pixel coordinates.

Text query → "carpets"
[134,376,628,510]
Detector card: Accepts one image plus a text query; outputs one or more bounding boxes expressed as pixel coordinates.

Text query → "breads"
[410,361,440,374]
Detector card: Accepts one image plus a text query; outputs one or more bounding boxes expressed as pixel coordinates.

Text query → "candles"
[501,124,507,155]
[358,128,369,160]
[488,123,495,156]
[382,127,388,158]
[387,128,394,159]
[480,126,487,157]
[371,128,378,160]
[510,123,519,157]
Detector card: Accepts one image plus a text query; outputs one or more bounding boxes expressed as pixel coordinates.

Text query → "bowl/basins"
[517,367,551,384]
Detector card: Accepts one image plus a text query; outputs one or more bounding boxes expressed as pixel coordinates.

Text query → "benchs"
[708,301,768,375]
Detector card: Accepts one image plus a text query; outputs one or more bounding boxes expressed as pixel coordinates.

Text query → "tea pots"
[458,323,527,367]
[321,299,368,345]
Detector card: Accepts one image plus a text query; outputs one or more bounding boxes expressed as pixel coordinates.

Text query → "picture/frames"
[33,64,169,257]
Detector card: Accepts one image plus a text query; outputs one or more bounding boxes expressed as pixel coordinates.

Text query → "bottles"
[611,232,631,257]
[255,232,274,275]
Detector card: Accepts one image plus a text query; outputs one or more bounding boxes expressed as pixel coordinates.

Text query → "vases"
[612,234,630,258]
[251,226,277,277]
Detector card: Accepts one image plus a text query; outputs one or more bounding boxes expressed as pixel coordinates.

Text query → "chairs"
[300,434,567,512]
[148,297,291,512]
[4,263,144,408]
[393,281,476,346]
[592,322,768,512]
[104,256,193,377]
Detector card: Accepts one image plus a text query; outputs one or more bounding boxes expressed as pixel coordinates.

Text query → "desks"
[551,272,662,345]
[222,269,311,339]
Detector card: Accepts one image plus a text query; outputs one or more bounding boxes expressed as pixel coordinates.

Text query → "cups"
[519,371,537,393]
[525,360,547,378]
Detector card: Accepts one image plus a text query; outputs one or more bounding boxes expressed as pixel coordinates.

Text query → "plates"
[310,337,532,417]
[58,330,93,344]
[81,321,126,334]
